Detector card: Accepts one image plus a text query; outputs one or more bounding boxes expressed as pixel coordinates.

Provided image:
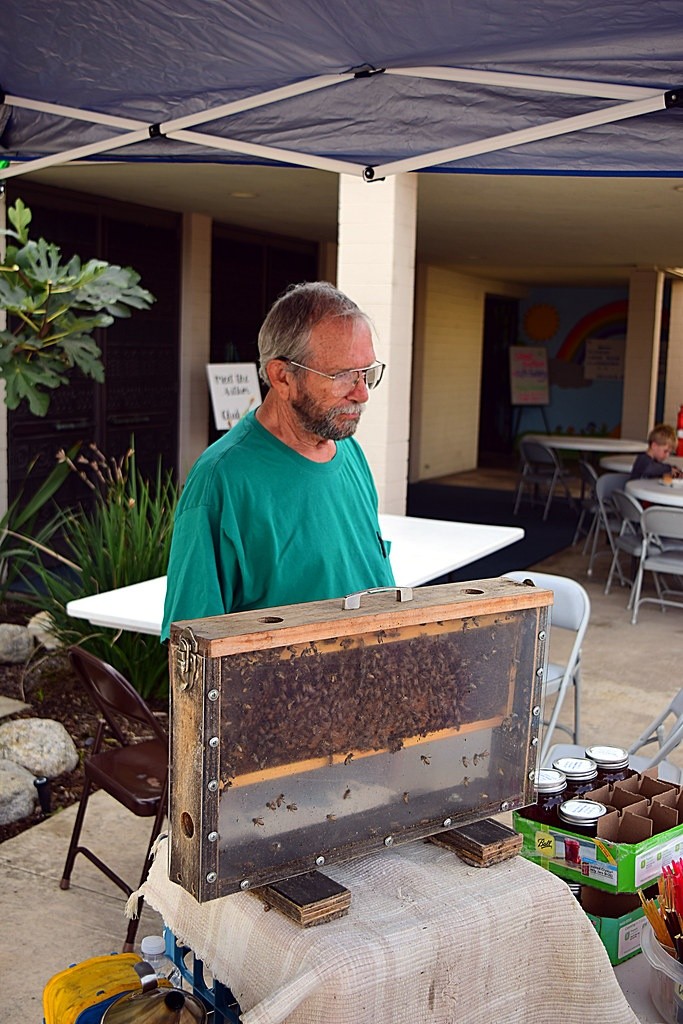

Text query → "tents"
[0,0,683,183]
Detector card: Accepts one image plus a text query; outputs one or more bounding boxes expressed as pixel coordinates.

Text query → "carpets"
[407,482,596,587]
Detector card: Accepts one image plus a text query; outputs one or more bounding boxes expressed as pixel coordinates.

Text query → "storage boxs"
[638,915,683,1024]
[512,775,683,886]
[566,888,658,969]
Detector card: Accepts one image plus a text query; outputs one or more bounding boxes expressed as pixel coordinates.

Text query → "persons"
[159,282,386,645]
[629,424,681,511]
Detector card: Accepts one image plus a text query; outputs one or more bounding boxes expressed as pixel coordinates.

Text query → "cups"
[585,744,629,786]
[558,800,606,839]
[552,757,598,800]
[518,768,568,828]
[564,838,579,861]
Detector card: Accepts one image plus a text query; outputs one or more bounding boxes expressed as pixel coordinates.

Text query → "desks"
[524,435,650,509]
[602,452,683,474]
[125,835,636,1024]
[67,515,523,664]
[625,479,683,590]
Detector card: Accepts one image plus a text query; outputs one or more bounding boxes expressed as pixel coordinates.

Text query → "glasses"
[275,355,386,398]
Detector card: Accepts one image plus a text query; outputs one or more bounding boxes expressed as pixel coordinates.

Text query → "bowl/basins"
[640,908,683,1024]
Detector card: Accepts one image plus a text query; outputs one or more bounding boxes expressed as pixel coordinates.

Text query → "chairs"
[62,646,169,953]
[572,459,599,555]
[501,572,590,766]
[604,490,643,594]
[590,473,630,588]
[512,439,573,521]
[631,507,683,626]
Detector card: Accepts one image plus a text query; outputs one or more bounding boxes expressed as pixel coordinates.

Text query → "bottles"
[140,934,182,989]
